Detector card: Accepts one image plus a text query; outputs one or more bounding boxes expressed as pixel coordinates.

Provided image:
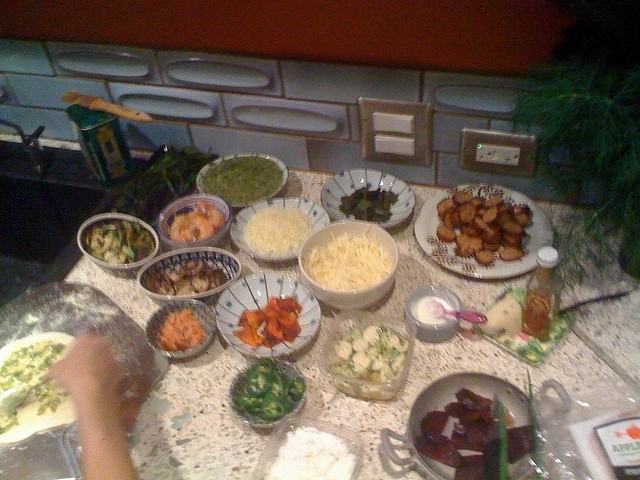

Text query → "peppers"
[229,357,306,423]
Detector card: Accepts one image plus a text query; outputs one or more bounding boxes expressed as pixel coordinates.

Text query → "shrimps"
[167,199,223,243]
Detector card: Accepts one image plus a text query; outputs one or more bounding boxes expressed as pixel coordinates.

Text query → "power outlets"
[461,127,538,178]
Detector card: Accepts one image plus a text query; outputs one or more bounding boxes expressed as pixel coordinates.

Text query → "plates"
[217,271,322,358]
[474,289,577,366]
[197,153,288,204]
[231,197,331,262]
[320,170,412,235]
[414,183,554,280]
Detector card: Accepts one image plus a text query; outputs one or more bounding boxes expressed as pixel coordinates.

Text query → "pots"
[379,372,573,480]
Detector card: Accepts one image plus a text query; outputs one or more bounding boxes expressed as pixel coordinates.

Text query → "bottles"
[521,247,561,343]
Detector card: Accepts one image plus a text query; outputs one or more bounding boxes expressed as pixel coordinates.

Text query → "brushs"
[62,88,154,124]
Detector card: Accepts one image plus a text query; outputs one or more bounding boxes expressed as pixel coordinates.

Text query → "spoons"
[423,303,487,326]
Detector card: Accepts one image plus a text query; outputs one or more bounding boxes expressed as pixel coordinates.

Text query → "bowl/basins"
[256,419,361,480]
[324,314,413,400]
[300,222,399,309]
[226,362,306,427]
[76,213,159,274]
[404,283,463,344]
[158,195,231,244]
[138,248,242,305]
[145,302,217,359]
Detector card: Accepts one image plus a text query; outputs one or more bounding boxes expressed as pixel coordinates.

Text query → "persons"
[48,332,139,480]
